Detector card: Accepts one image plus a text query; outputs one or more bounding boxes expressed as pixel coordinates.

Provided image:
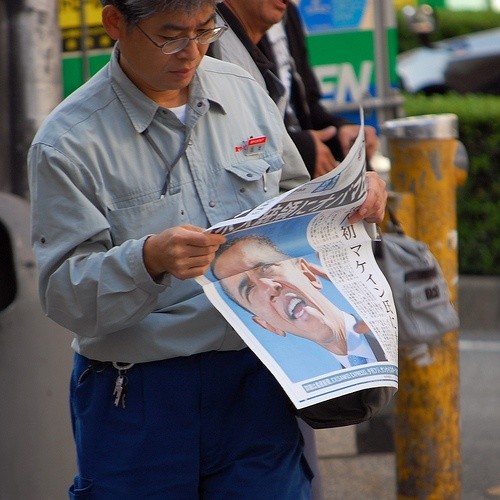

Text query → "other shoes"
[354,411,402,452]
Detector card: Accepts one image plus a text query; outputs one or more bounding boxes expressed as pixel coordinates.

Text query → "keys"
[110,373,131,411]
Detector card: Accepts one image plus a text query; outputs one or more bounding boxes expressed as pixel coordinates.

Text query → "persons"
[27,0,389,500]
[210,234,388,370]
[214,0,379,182]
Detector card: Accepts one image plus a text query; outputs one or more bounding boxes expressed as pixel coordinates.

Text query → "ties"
[348,353,368,370]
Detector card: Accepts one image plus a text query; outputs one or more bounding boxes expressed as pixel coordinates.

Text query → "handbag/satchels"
[368,198,462,347]
[296,383,391,432]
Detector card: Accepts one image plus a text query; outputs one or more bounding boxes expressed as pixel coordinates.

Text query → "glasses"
[131,4,230,57]
